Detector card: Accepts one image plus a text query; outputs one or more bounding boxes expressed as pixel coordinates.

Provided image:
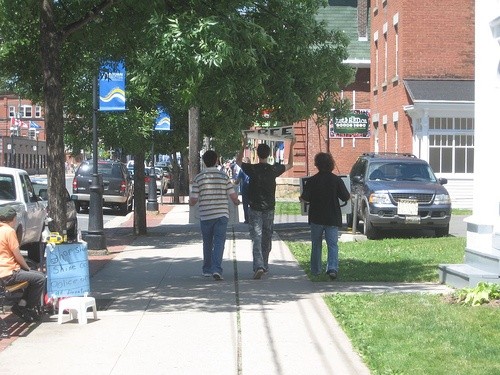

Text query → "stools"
[57,296,98,324]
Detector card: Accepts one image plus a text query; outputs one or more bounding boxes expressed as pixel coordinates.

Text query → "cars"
[31,178,80,245]
[126,156,181,197]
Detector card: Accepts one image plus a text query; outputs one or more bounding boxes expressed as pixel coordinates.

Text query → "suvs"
[70,160,134,216]
[350,152,453,239]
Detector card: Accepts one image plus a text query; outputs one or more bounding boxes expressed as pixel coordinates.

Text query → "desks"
[39,238,91,314]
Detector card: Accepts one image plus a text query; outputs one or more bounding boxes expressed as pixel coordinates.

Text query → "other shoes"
[213,273,223,281]
[25,306,40,321]
[327,269,337,280]
[203,272,212,277]
[244,221,249,224]
[253,268,264,279]
[10,304,32,325]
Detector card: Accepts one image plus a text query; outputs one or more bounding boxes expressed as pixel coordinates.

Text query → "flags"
[30,120,41,128]
[12,118,21,126]
[20,121,28,128]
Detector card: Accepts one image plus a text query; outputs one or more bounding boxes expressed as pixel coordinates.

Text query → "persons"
[217,158,241,180]
[300,152,350,281]
[236,138,296,279]
[188,151,240,281]
[0,206,46,322]
[234,157,252,224]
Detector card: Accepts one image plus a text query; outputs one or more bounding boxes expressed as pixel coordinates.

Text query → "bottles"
[62,228,68,244]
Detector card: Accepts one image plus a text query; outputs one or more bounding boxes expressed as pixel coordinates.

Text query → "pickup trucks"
[1,166,48,262]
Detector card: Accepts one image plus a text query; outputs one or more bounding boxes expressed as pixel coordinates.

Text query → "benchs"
[0,257,38,342]
